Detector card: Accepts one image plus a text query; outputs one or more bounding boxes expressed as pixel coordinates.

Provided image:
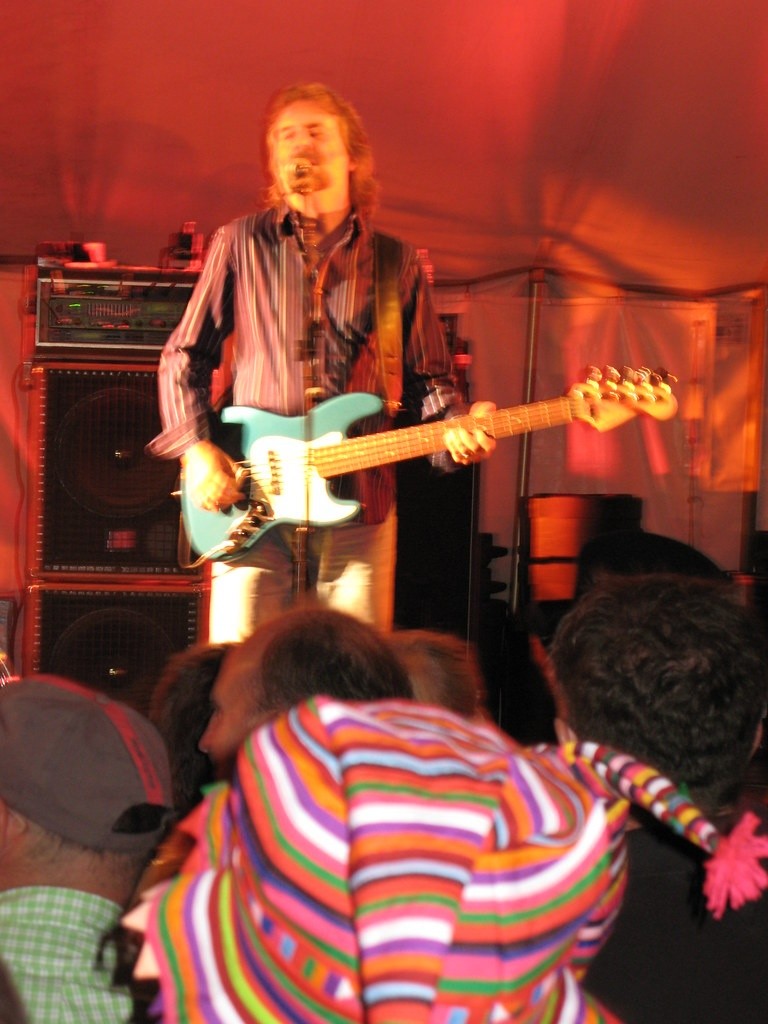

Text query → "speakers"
[25,361,207,582]
[22,580,210,722]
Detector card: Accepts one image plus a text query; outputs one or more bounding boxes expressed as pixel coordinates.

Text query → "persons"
[151,642,230,815]
[111,690,768,1024]
[382,627,493,723]
[548,574,768,1024]
[197,603,415,784]
[142,82,497,648]
[0,670,181,1024]
[45,608,179,713]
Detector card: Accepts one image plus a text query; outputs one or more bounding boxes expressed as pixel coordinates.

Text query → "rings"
[463,454,470,458]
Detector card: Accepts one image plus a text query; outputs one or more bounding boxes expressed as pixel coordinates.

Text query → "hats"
[0,672,183,854]
[119,694,768,1024]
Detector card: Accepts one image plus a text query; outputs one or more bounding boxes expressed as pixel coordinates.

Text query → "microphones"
[288,157,314,178]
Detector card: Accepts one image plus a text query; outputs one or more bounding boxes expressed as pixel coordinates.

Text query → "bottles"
[417,248,434,295]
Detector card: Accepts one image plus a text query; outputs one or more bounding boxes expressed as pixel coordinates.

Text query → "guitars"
[180,364,681,567]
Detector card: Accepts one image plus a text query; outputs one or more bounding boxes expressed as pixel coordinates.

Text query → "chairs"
[498,492,645,744]
